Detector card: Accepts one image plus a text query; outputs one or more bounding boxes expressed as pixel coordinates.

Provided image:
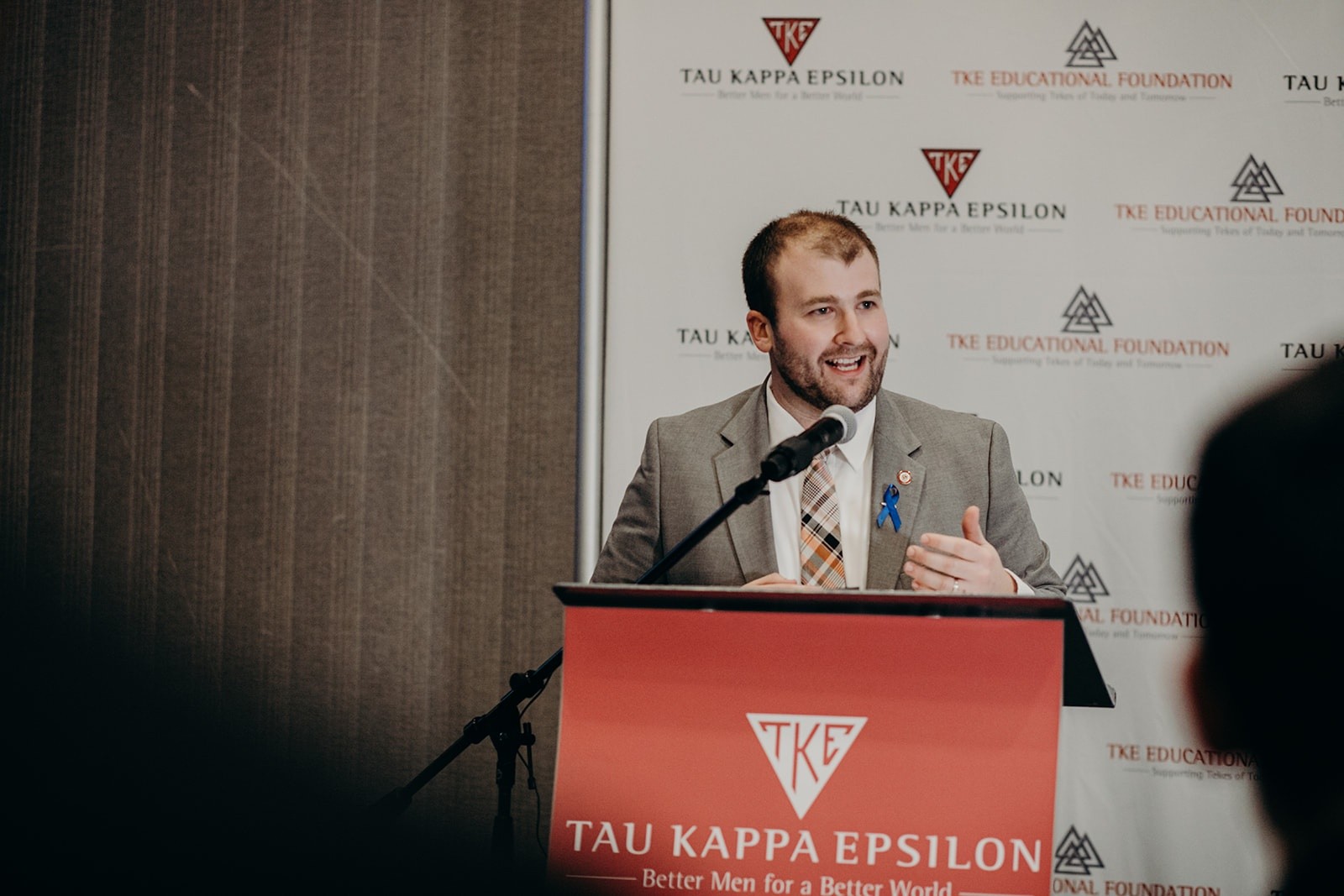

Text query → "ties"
[800,445,846,589]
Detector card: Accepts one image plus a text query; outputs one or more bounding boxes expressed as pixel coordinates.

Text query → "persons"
[1174,345,1344,896]
[585,202,1069,598]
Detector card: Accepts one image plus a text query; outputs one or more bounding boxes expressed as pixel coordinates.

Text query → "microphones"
[760,404,857,483]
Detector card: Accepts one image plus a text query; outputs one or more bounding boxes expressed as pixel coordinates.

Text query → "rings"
[950,579,959,593]
